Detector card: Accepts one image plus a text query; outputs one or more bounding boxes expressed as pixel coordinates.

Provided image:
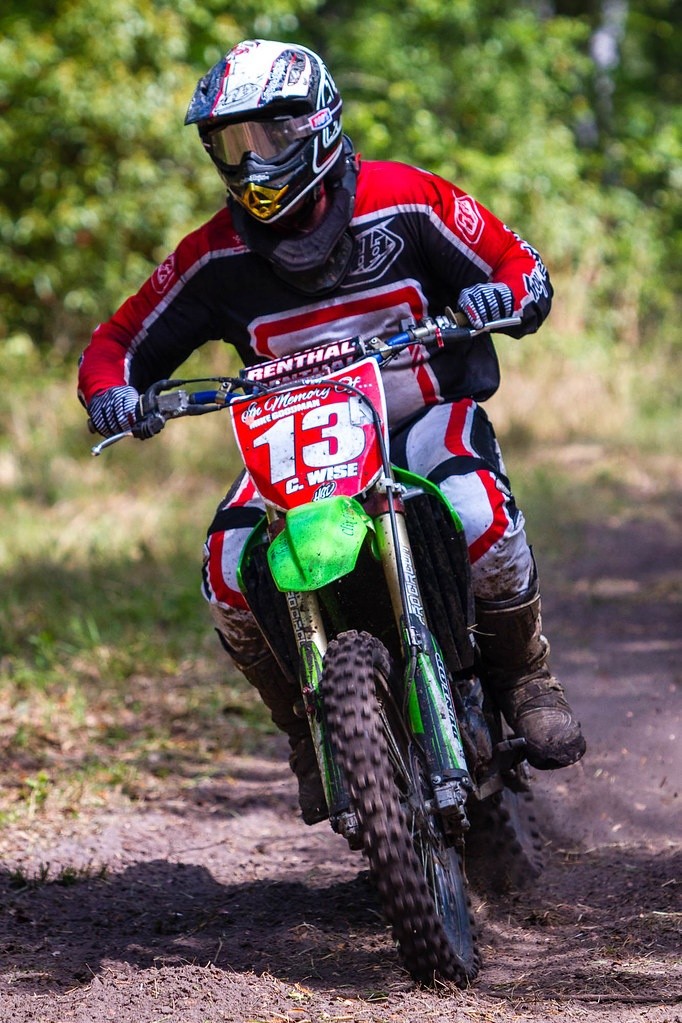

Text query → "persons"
[78,37,588,827]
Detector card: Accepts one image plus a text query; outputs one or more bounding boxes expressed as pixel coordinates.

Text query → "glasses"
[208,115,304,173]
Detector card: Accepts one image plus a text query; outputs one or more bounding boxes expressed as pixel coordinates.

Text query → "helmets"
[184,39,342,224]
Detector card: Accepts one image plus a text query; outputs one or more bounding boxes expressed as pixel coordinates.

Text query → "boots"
[213,627,329,826]
[474,544,587,771]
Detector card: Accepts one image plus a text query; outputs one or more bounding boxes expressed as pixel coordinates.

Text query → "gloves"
[87,385,139,439]
[457,282,514,330]
